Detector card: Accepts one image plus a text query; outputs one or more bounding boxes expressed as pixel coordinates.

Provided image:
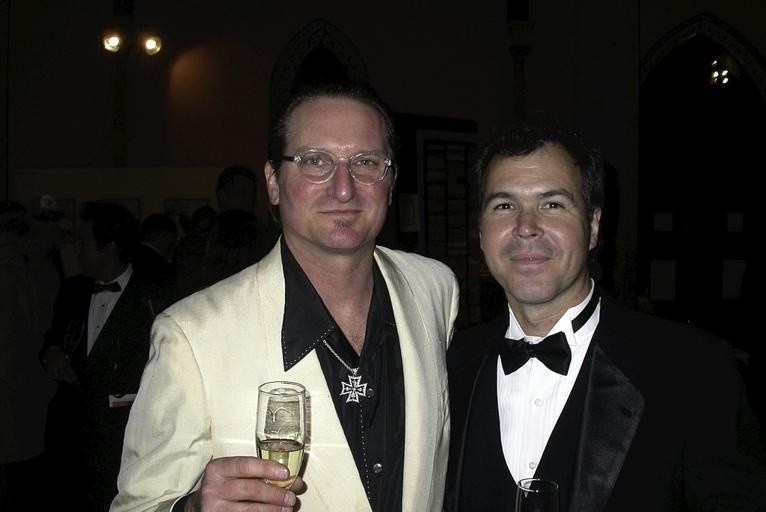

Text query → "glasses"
[278,151,394,186]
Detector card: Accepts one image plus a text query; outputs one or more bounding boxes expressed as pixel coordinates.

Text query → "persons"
[444,116,765,512]
[1,165,277,510]
[108,88,460,512]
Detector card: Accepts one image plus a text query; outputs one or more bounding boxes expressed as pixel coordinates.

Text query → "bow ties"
[90,281,122,293]
[498,331,571,375]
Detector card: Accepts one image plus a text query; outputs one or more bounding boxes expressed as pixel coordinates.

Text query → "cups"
[515,477,561,512]
[254,381,307,491]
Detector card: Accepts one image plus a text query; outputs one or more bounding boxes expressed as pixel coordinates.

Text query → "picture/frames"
[32,198,76,224]
[165,199,209,220]
[96,199,141,223]
